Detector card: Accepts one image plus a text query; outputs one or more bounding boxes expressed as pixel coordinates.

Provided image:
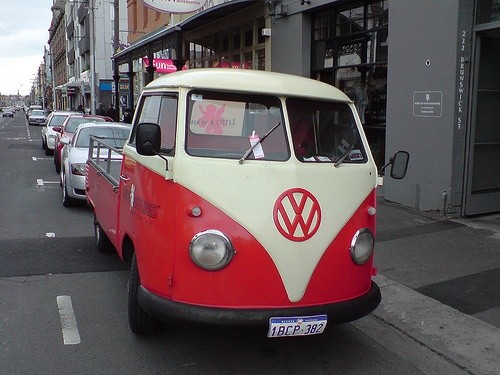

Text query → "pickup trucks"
[83,66,410,337]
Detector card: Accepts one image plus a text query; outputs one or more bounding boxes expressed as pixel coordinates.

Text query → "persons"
[47,102,53,114]
[77,105,85,114]
[106,103,116,121]
[124,109,132,123]
[346,77,368,124]
[96,103,105,116]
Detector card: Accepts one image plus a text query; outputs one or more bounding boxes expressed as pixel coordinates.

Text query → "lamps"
[267,0,288,24]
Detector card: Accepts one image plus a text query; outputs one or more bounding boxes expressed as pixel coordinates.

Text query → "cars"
[0,104,49,126]
[39,110,84,156]
[51,114,115,175]
[58,122,133,208]
